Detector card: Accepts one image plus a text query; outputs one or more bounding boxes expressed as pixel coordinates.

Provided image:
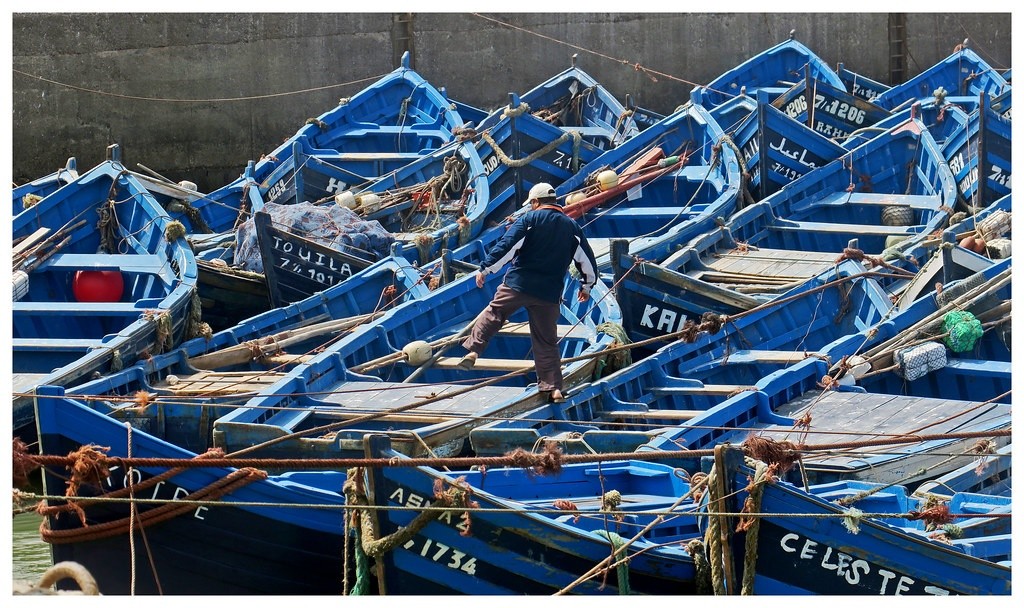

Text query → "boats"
[169,49,490,311]
[11,142,196,443]
[11,30,1010,596]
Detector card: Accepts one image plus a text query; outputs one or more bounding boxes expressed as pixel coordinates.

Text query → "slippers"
[547,391,567,403]
[456,356,476,371]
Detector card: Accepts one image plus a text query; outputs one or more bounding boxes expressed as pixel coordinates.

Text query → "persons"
[458,183,598,403]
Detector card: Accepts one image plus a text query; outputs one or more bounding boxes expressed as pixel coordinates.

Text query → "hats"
[521,182,556,206]
